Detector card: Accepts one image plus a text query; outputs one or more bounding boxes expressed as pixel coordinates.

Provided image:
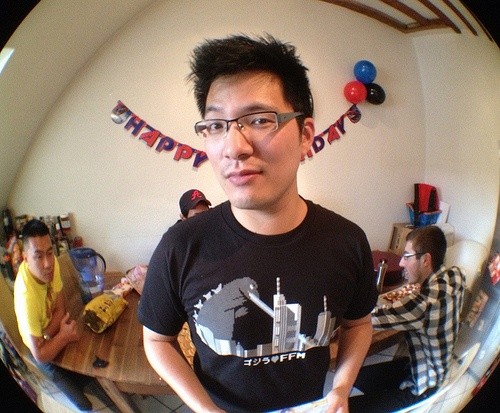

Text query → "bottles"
[377,259,388,293]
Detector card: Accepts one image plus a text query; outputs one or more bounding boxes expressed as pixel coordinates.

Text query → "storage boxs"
[406,202,441,227]
[390,222,454,262]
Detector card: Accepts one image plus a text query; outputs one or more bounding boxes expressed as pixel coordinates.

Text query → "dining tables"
[370,250,403,290]
[49,272,197,413]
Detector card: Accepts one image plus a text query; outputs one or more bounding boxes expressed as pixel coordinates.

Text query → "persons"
[347,225,465,413]
[13,219,93,411]
[176,188,211,223]
[137,30,379,413]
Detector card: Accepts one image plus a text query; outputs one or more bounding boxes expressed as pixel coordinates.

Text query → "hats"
[179,188,212,210]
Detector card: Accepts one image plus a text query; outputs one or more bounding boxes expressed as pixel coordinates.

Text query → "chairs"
[443,239,489,312]
[0,271,54,384]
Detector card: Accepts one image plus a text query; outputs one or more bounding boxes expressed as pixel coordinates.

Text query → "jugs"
[69,247,106,304]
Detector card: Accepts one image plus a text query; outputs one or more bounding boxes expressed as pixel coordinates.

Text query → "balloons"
[343,60,386,105]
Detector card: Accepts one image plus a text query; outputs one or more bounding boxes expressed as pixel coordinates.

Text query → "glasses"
[403,252,422,261]
[193,110,306,141]
